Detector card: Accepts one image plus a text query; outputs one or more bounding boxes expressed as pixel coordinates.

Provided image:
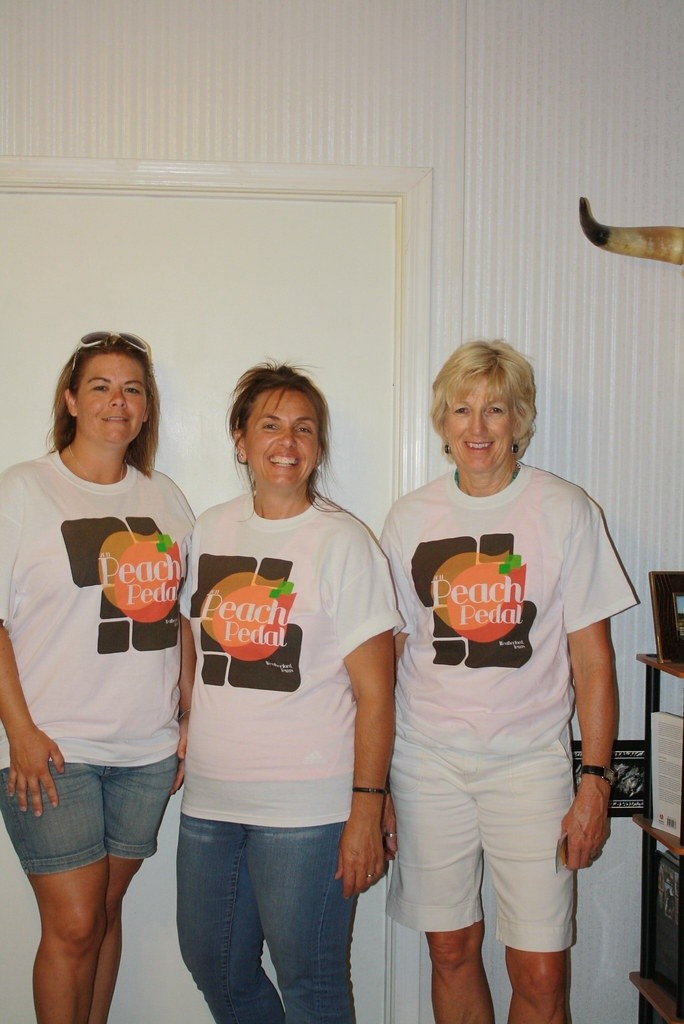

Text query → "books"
[649,710,684,838]
[654,849,679,1001]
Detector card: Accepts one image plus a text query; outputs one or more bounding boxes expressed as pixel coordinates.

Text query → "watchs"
[580,763,615,785]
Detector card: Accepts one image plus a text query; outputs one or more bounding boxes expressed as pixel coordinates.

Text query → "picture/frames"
[650,571,684,664]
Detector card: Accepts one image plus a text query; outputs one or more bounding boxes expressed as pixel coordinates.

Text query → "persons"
[372,341,640,1023]
[0,332,200,1022]
[175,358,404,1023]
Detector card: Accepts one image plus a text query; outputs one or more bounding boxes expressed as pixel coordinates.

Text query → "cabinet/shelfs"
[628,653,684,1023]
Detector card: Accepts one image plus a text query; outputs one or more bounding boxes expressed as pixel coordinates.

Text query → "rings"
[366,873,375,878]
[385,832,397,839]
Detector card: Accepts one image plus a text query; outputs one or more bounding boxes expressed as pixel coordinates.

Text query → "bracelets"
[352,785,389,795]
[178,706,191,719]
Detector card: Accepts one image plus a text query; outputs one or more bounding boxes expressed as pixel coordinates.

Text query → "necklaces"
[454,466,521,497]
[66,443,125,486]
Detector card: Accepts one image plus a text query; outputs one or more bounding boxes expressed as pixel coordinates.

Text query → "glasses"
[71,330,152,372]
